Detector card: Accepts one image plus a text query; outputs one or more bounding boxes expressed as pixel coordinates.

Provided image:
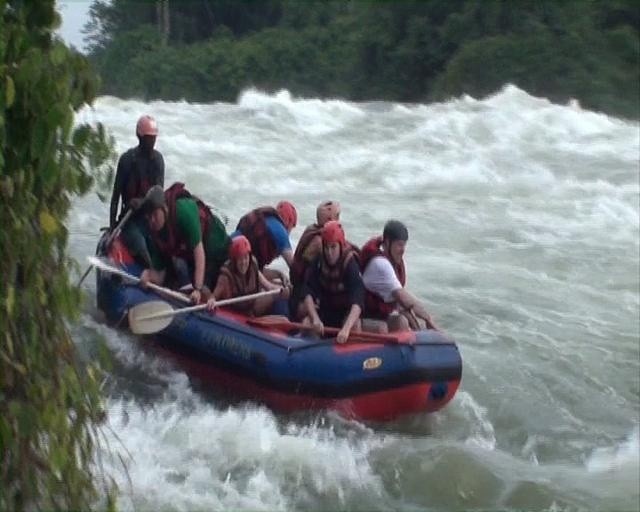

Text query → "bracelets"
[193,286,202,291]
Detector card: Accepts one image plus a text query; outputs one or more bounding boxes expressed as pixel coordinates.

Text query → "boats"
[95,245,464,421]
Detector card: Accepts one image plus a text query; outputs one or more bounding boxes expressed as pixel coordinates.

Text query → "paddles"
[245,312,416,347]
[129,287,281,333]
[86,255,192,304]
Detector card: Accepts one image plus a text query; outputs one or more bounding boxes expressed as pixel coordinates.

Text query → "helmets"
[229,235,251,257]
[277,202,297,228]
[136,116,159,136]
[316,201,345,253]
[142,185,167,209]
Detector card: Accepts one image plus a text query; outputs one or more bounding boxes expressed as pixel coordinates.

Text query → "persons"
[206,236,289,318]
[358,219,440,335]
[109,115,165,270]
[301,219,367,345]
[139,181,232,307]
[290,199,361,323]
[227,199,297,274]
